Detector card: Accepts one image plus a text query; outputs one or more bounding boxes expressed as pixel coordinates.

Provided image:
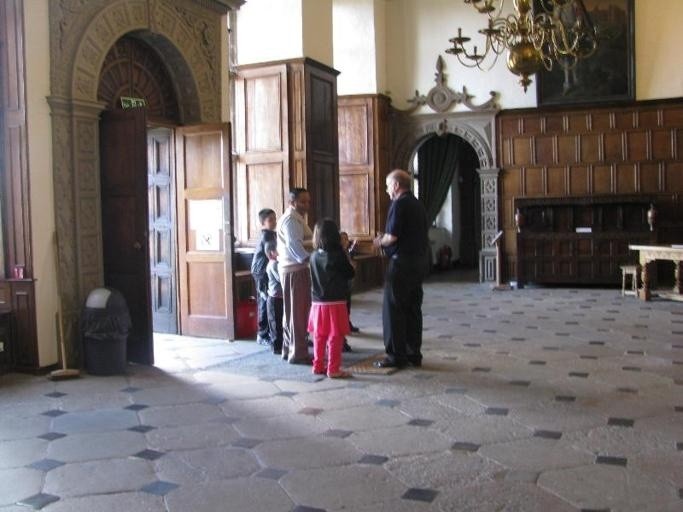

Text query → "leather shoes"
[403,355,423,368]
[371,353,400,369]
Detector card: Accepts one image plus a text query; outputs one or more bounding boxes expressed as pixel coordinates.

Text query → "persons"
[250,168,427,377]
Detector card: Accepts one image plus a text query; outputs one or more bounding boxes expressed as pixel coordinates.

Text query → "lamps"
[443,0,599,95]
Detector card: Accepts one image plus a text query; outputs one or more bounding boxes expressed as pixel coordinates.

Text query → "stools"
[619,265,640,299]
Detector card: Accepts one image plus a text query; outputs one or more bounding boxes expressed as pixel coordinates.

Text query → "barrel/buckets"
[234,300,258,338]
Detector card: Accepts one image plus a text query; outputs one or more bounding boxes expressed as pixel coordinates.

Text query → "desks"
[627,247,682,302]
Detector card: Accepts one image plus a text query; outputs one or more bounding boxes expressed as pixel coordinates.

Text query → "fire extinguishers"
[440,245,449,271]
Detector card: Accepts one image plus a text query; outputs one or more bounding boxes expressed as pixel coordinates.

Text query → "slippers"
[313,368,327,374]
[327,370,354,378]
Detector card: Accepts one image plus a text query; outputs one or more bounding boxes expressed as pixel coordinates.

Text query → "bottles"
[647,204,656,231]
[513,207,524,233]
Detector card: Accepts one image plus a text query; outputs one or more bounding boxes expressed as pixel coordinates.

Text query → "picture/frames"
[532,0,636,107]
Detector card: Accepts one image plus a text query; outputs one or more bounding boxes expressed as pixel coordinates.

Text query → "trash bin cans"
[77,287,133,376]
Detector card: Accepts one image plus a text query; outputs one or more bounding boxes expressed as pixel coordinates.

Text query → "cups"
[13,264,23,279]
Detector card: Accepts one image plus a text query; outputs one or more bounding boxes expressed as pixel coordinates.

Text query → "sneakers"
[256,334,272,346]
[288,354,314,365]
[348,322,359,333]
[273,348,282,354]
[281,353,290,361]
